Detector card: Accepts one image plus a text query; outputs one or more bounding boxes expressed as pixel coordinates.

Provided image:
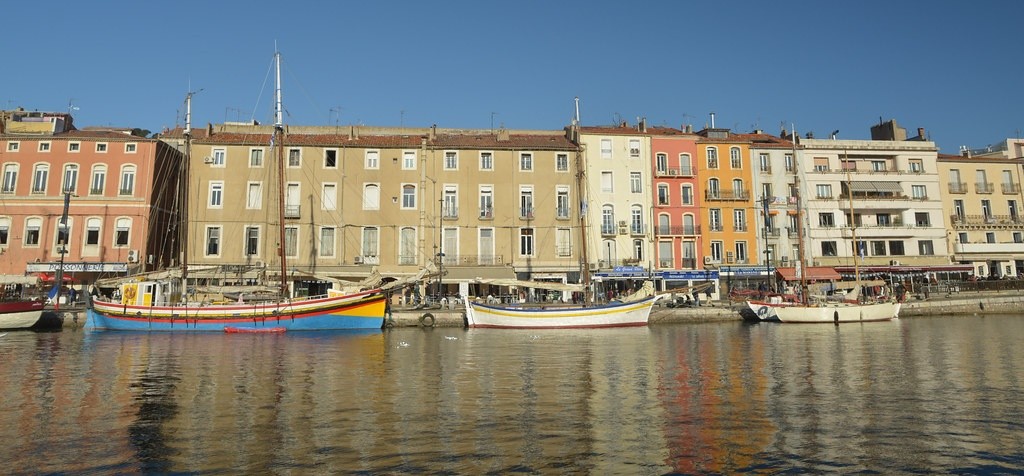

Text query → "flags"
[48,285,58,311]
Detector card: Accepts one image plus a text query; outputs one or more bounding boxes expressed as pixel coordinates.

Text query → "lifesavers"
[421,313,435,327]
[125,287,135,299]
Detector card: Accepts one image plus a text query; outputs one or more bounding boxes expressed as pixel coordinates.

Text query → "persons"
[758,281,767,300]
[896,281,906,302]
[705,286,713,306]
[67,288,77,305]
[607,288,632,301]
[923,275,928,286]
[692,287,701,306]
[874,277,881,295]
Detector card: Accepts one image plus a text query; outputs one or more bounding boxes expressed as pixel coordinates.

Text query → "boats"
[0,185,45,329]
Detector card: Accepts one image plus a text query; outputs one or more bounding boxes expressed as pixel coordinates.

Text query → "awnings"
[42,273,76,288]
[777,267,841,280]
[26,261,127,273]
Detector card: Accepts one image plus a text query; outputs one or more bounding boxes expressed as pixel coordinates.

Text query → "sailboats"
[735,124,902,323]
[464,98,713,329]
[84,38,449,331]
